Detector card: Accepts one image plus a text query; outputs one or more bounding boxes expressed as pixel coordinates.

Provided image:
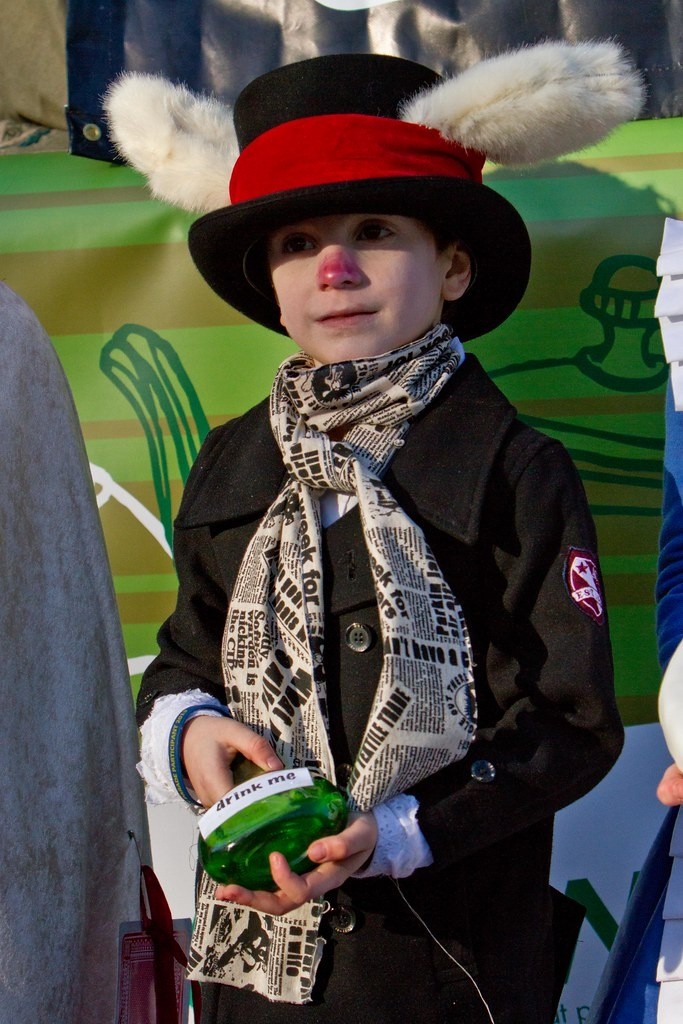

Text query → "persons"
[135,49,624,1024]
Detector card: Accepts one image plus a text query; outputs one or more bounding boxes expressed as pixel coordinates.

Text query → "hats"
[100,35,647,343]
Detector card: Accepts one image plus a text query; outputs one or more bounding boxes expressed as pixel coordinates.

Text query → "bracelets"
[171,704,239,807]
